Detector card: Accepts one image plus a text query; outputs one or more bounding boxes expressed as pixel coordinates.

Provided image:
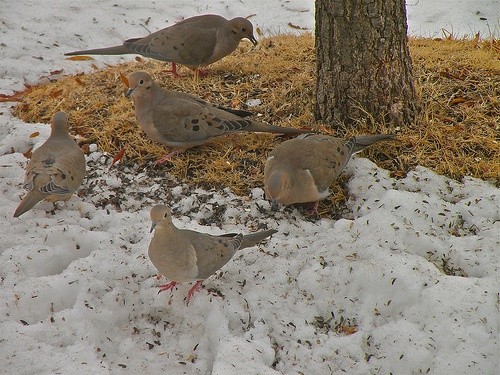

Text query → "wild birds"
[12,112,86,218]
[64,14,257,80]
[124,72,313,166]
[147,205,278,307]
[264,133,392,217]
